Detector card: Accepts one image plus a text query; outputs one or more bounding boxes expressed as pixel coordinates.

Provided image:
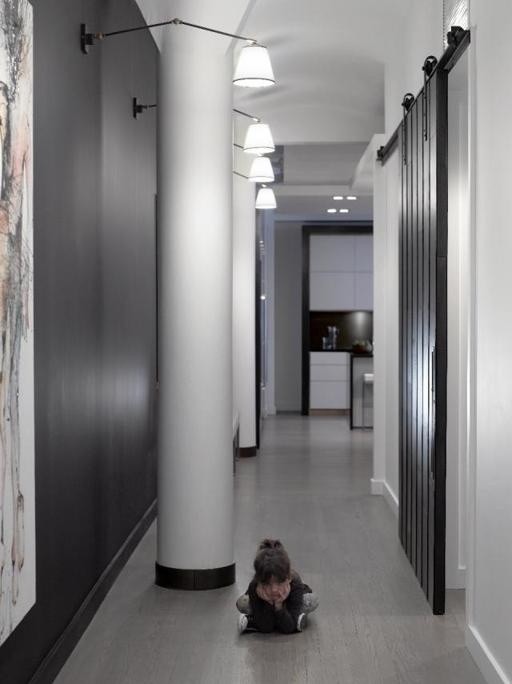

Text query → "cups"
[323,326,340,349]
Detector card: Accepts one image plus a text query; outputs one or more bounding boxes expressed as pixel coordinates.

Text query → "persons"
[235,537,319,636]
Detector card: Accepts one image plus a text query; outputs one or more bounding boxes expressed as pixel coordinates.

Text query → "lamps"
[81,18,280,212]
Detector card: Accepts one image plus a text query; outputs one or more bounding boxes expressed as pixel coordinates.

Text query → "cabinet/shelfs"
[301,223,376,430]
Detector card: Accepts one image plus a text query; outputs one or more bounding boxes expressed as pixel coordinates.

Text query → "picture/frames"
[1,0,36,648]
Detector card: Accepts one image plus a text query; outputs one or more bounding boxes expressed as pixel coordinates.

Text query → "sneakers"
[237,612,249,634]
[296,611,308,632]
